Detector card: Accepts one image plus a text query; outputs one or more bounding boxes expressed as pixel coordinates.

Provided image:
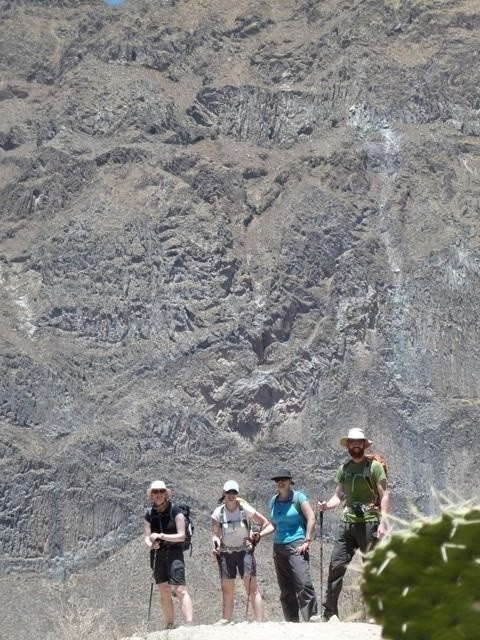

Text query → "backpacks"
[177,504,194,552]
[365,453,388,481]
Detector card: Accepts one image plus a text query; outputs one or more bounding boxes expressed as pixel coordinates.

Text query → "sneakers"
[309,614,329,623]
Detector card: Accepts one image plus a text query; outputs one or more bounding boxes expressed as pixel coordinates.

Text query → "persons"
[310,427,390,623]
[144,480,194,630]
[251,469,317,622]
[210,480,269,625]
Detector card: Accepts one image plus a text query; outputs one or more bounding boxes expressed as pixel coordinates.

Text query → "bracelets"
[160,532,164,539]
[304,539,313,543]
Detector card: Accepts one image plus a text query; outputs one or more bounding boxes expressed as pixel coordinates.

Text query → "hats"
[146,480,171,498]
[223,480,240,495]
[271,468,292,480]
[339,428,373,448]
